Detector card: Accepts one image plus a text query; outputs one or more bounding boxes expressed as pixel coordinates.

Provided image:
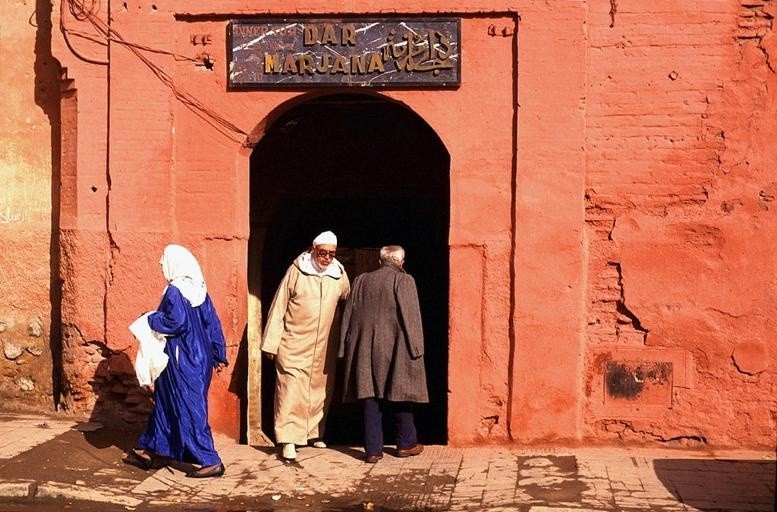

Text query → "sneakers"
[130,448,153,467]
[367,453,383,463]
[283,442,297,459]
[187,463,224,477]
[397,443,423,457]
[311,439,326,448]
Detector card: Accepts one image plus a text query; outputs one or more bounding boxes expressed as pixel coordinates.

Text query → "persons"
[257,229,352,462]
[336,244,429,463]
[127,242,230,478]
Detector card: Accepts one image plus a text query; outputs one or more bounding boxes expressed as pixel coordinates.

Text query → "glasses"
[314,246,337,259]
